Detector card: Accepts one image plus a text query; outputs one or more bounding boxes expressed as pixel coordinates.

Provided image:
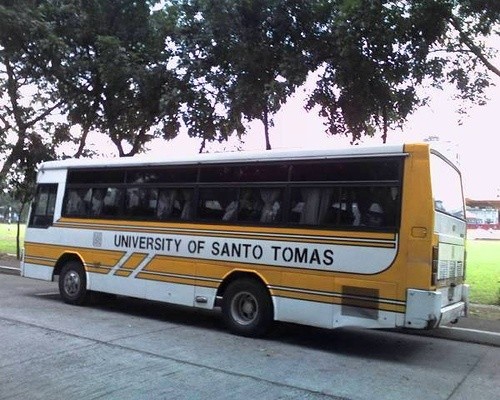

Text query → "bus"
[19,143,468,341]
